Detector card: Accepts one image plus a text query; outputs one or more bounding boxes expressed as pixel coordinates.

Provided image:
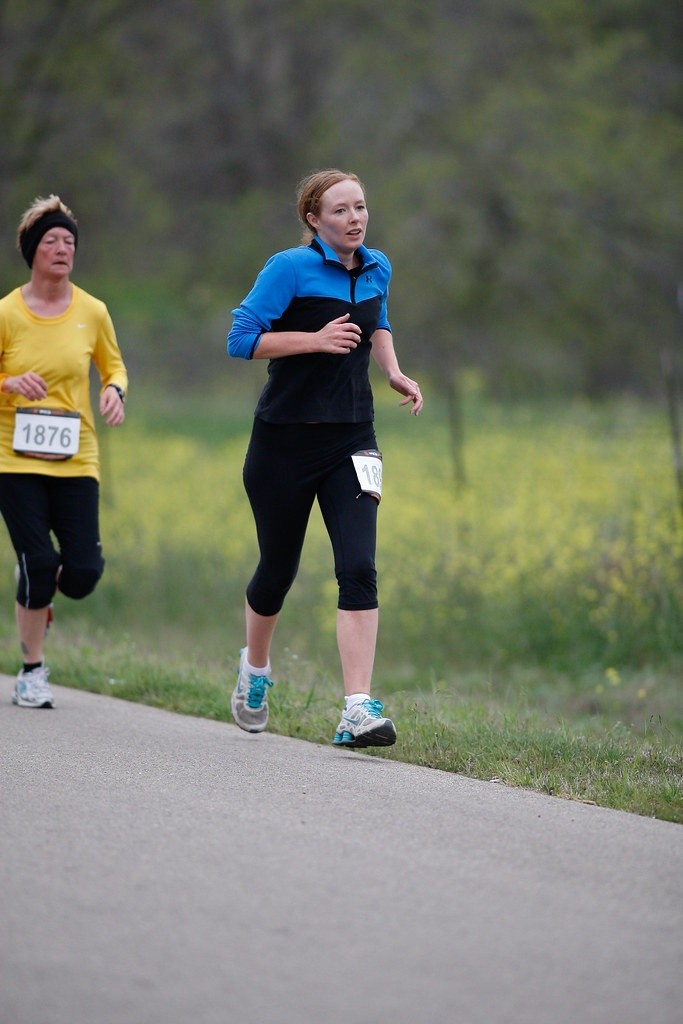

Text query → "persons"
[227,169,423,746]
[0,194,129,710]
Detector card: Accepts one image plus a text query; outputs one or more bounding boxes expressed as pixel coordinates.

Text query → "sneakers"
[13,666,57,709]
[334,701,397,750]
[14,564,53,641]
[230,647,275,733]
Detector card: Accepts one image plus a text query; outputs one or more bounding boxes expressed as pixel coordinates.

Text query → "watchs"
[107,382,125,402]
